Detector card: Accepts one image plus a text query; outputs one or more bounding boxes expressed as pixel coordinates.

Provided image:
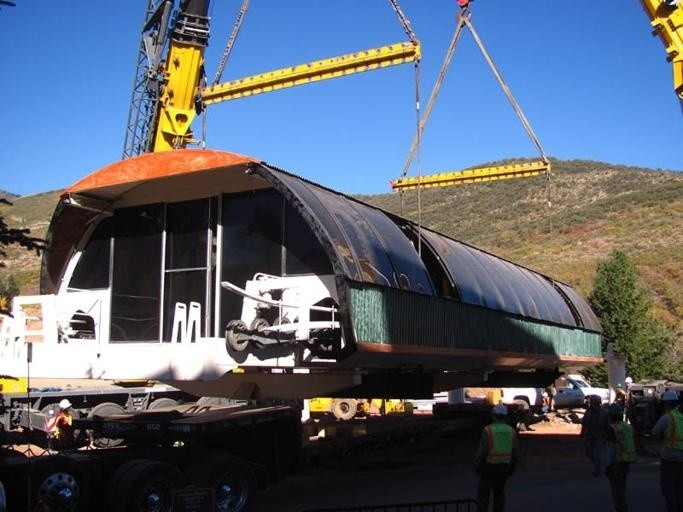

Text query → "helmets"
[491,403,508,416]
[663,390,678,400]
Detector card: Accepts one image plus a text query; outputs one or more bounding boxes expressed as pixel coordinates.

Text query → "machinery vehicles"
[0,0,682,452]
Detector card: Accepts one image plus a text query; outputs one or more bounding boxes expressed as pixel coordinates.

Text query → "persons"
[597,403,638,510]
[473,403,521,512]
[576,393,607,475]
[55,397,77,450]
[649,388,682,512]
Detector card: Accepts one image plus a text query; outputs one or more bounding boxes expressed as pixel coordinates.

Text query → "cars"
[553,374,610,405]
